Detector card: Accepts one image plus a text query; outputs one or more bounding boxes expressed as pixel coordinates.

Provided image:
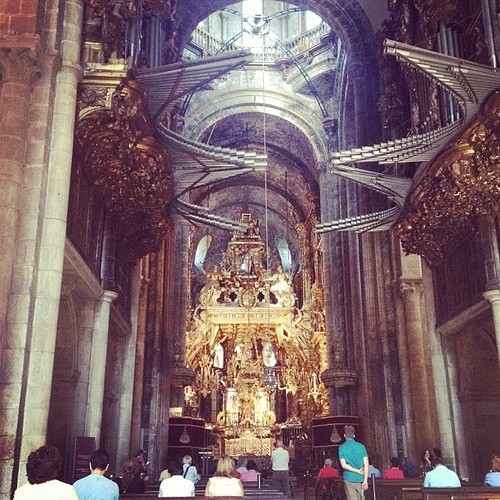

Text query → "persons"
[159,469,172,484]
[241,460,261,482]
[13,445,79,500]
[236,459,248,474]
[338,426,369,500]
[369,460,381,477]
[122,449,150,494]
[383,457,404,479]
[73,448,119,500]
[158,462,195,497]
[483,454,500,487]
[205,455,244,496]
[270,441,289,496]
[419,449,432,472]
[182,455,197,487]
[318,458,339,500]
[202,243,295,421]
[422,448,461,488]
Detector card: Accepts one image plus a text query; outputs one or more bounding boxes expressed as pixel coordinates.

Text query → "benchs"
[115,469,500,500]
